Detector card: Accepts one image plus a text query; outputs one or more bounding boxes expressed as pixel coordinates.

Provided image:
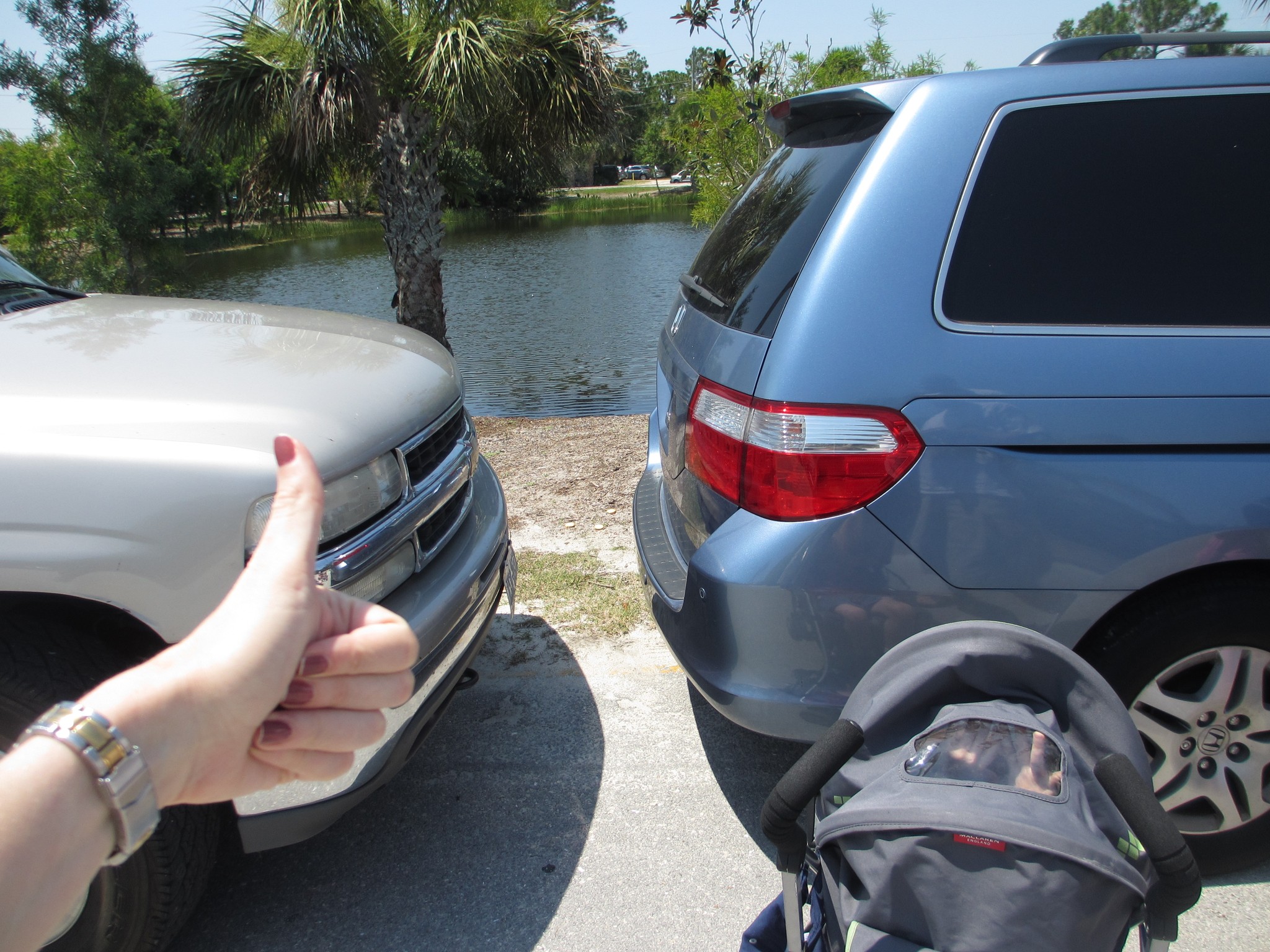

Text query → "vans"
[595,165,619,186]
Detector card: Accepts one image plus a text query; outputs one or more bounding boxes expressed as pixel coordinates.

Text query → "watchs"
[10,701,159,865]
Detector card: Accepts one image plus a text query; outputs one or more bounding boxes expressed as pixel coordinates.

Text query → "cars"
[617,166,626,182]
[670,169,713,183]
[647,164,665,178]
[624,165,653,180]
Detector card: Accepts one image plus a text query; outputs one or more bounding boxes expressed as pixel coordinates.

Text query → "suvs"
[628,26,1270,878]
[0,252,522,951]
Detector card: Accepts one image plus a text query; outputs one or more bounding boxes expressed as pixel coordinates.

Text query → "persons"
[947,721,1063,798]
[0,432,419,952]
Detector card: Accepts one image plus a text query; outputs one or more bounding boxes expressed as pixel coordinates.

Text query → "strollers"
[741,618,1203,952]
